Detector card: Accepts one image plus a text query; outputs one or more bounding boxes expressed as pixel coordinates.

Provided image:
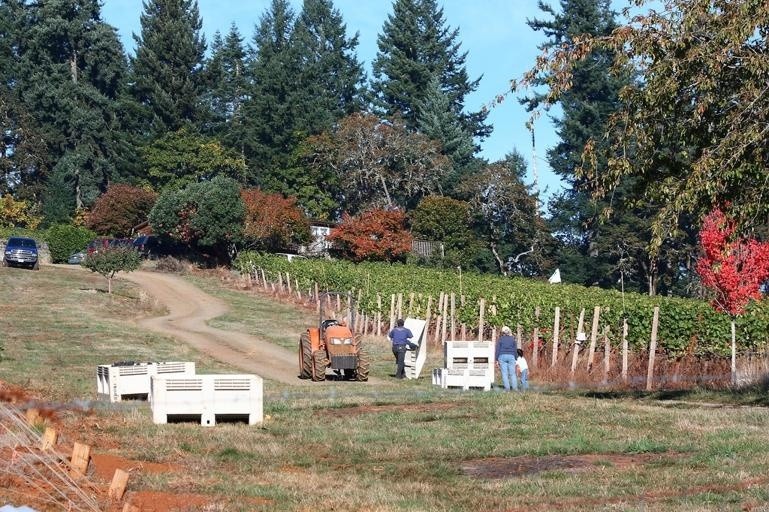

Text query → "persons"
[389,318,414,378]
[496,325,519,391]
[514,348,530,390]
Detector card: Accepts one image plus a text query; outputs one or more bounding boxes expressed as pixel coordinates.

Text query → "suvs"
[3,236,39,272]
[275,252,309,266]
[66,232,180,266]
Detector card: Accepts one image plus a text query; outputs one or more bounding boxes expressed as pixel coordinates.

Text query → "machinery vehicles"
[298,288,370,383]
